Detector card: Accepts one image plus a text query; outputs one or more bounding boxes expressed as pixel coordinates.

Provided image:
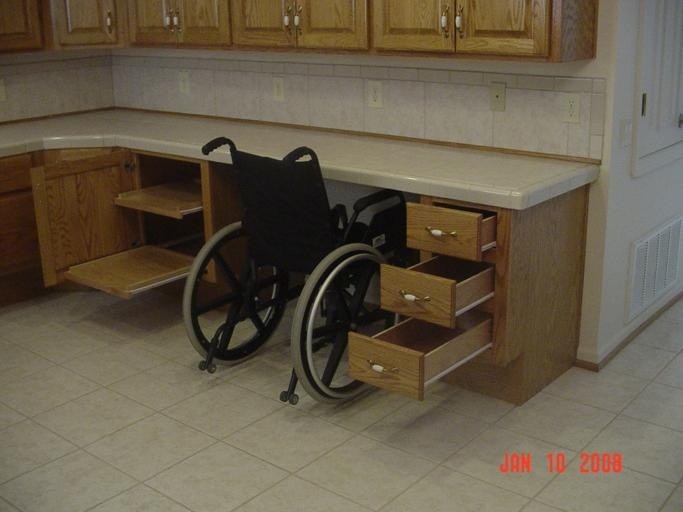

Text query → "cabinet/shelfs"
[0,148,270,408]
[345,183,591,407]
[0,0,599,64]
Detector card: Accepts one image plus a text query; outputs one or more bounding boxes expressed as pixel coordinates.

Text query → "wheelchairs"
[182,137,413,404]
